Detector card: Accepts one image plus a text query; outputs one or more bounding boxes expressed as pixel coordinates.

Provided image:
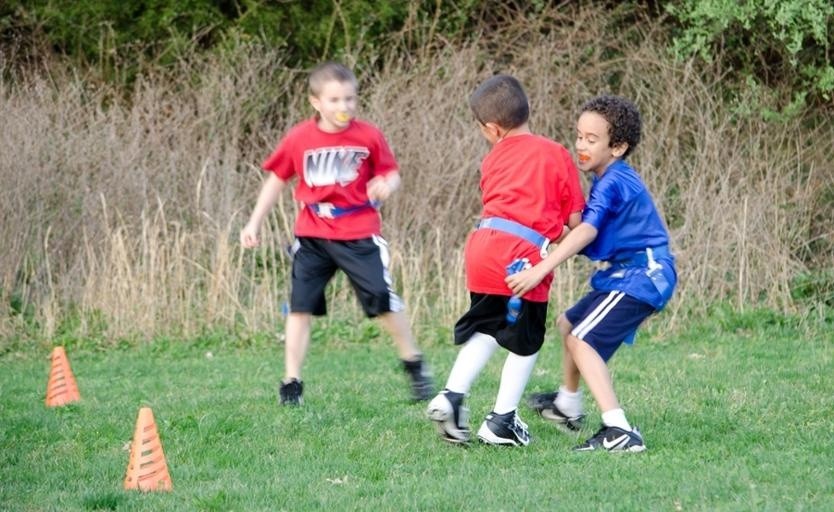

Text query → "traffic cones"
[46,346,80,407]
[124,407,172,492]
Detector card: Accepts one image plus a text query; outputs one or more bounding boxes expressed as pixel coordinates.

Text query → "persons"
[240,60,435,405]
[423,73,583,449]
[505,97,678,453]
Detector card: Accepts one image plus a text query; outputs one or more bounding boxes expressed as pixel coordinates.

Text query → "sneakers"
[572,427,646,453]
[412,377,434,398]
[427,388,472,442]
[533,391,585,430]
[278,378,302,408]
[477,408,532,447]
